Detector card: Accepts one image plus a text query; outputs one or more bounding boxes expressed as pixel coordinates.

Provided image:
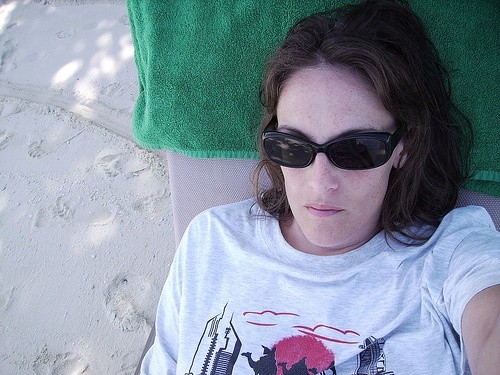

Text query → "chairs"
[127,0,500,200]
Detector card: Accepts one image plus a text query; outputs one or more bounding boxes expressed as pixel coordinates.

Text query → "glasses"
[261,116,407,170]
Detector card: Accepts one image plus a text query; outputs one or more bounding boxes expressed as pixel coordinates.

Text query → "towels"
[125,0,498,197]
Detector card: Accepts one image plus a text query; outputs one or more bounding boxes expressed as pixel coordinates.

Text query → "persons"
[140,0,500,375]
